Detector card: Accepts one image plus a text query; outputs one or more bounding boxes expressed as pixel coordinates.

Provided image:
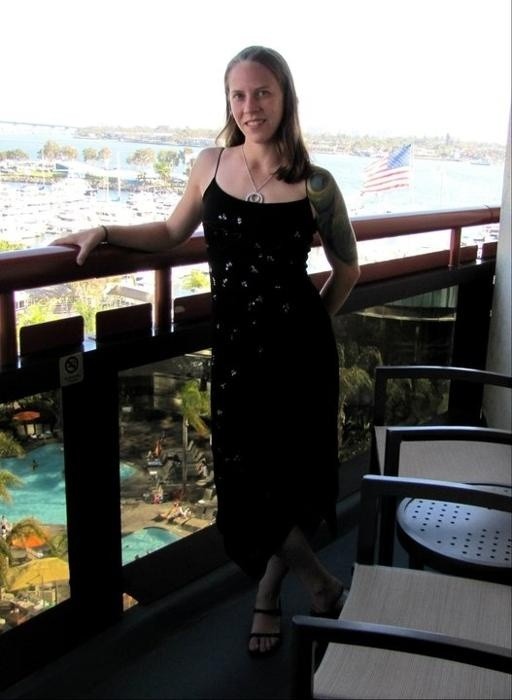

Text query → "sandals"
[248,593,284,658]
[307,586,350,619]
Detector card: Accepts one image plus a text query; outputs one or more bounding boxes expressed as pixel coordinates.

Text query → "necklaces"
[238,136,284,204]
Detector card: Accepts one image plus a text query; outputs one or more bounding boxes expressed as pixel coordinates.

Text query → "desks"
[291,474,511,700]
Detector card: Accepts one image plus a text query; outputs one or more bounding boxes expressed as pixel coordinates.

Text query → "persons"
[196,461,208,479]
[47,44,361,661]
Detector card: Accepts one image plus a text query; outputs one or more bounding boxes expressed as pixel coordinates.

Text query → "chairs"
[356,365,512,567]
[399,480,511,584]
[10,597,48,625]
[146,440,217,527]
[23,547,44,563]
[15,422,54,446]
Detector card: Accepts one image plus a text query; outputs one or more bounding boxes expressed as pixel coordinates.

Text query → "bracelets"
[101,225,110,243]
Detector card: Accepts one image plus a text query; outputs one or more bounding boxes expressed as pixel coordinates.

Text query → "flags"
[361,143,410,194]
